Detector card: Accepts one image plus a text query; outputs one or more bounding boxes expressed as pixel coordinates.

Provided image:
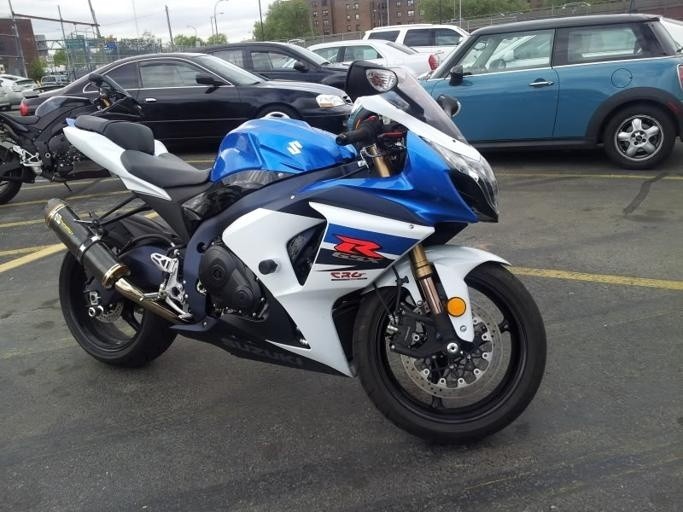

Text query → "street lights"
[183,0,230,48]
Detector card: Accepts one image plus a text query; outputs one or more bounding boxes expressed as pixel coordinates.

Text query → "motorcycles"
[39,59,547,445]
[0,73,147,201]
